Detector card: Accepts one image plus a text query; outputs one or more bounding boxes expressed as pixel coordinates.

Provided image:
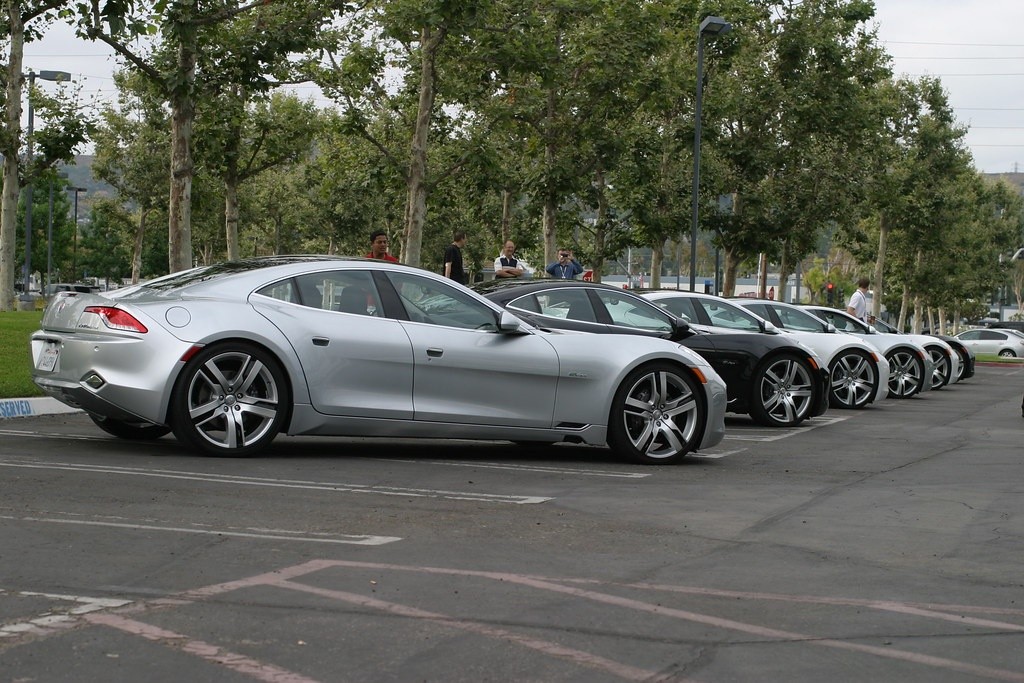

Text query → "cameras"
[560,252,569,257]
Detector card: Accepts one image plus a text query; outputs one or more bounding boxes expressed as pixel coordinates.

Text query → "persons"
[442,230,465,285]
[493,240,523,279]
[364,230,399,304]
[545,248,583,279]
[845,278,870,331]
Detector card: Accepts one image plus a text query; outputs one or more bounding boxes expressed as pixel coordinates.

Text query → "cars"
[699,295,891,410]
[18,284,101,299]
[949,321,1024,359]
[772,304,981,399]
[417,279,832,429]
[30,258,727,467]
[543,287,779,339]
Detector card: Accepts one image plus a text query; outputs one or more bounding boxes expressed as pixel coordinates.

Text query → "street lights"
[689,17,726,292]
[23,71,69,293]
[67,185,87,281]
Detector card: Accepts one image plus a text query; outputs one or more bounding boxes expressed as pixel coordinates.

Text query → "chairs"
[339,286,371,315]
[663,304,682,320]
[566,299,596,321]
[291,281,325,306]
